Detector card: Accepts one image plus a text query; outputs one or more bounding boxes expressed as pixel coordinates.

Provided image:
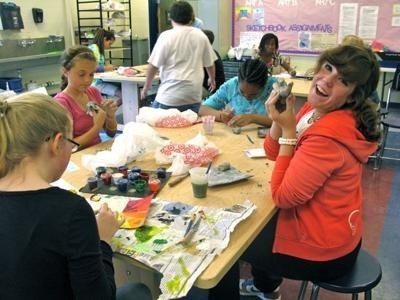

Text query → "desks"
[55,121,280,300]
[91,65,160,124]
[272,72,313,102]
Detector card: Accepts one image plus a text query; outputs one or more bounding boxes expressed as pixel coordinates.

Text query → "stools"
[377,115,400,168]
[297,249,382,300]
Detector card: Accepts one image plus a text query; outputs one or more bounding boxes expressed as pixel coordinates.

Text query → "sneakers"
[239,277,282,300]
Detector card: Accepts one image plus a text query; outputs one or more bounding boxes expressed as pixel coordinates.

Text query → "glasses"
[45,131,81,153]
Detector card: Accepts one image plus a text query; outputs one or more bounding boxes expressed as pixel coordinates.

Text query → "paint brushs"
[186,216,202,241]
[206,161,212,174]
[94,209,100,214]
[172,238,207,254]
[149,240,184,261]
[184,213,197,238]
[246,134,254,144]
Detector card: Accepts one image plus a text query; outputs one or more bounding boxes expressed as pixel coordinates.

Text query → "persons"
[87,29,116,73]
[0,93,155,300]
[342,35,380,112]
[239,45,381,300]
[201,30,225,99]
[53,47,118,152]
[198,59,279,128]
[254,33,296,76]
[140,1,219,113]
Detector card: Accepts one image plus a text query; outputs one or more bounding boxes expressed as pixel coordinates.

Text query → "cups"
[189,167,211,198]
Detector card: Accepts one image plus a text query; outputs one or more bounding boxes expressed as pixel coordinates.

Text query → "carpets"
[239,162,393,300]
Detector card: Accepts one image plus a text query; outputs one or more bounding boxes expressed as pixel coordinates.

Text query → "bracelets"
[106,119,117,131]
[279,137,298,147]
